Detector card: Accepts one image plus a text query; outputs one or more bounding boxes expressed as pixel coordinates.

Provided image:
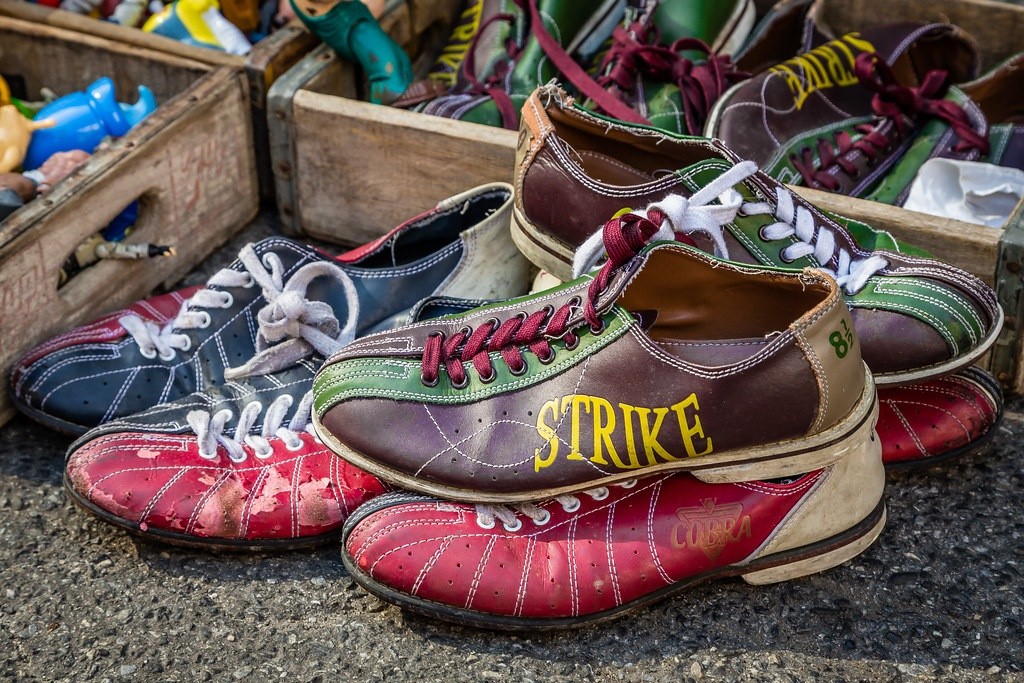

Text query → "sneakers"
[876,365,1005,468]
[62,360,405,552]
[510,83,1004,389]
[342,431,887,630]
[390,0,1024,208]
[312,244,880,503]
[11,182,532,434]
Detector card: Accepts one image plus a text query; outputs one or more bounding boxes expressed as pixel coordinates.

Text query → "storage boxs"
[0,13,257,426]
[0,0,324,192]
[266,0,1024,392]
